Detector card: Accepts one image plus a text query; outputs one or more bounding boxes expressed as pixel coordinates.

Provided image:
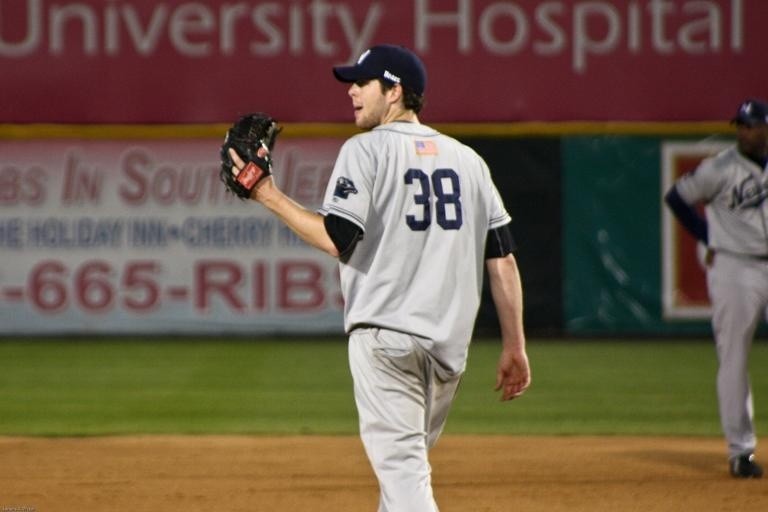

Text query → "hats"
[332,46,426,95]
[731,99,768,131]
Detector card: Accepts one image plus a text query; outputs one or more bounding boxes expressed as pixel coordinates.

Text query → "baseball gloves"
[220,112,283,201]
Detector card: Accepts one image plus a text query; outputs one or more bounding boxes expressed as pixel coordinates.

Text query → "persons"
[663,99,768,478]
[222,43,532,512]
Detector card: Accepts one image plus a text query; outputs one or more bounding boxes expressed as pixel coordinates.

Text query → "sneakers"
[730,449,763,479]
